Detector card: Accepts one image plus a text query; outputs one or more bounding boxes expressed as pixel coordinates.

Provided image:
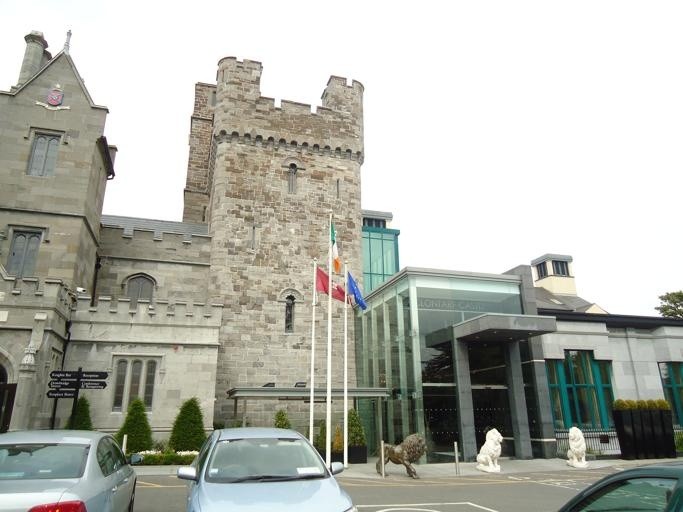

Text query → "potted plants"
[609,395,680,460]
[345,406,369,464]
[328,421,344,463]
[311,419,326,463]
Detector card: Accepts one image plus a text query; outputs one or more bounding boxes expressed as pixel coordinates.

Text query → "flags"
[317,267,352,304]
[347,272,367,310]
[331,222,341,274]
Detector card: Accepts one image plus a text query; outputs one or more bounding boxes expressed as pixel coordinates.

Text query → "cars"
[173,426,356,511]
[557,461,683,512]
[0,429,144,512]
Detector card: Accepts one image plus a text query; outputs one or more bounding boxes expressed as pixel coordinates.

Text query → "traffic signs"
[45,390,75,397]
[79,381,107,389]
[49,370,79,378]
[46,380,78,389]
[80,371,107,380]
